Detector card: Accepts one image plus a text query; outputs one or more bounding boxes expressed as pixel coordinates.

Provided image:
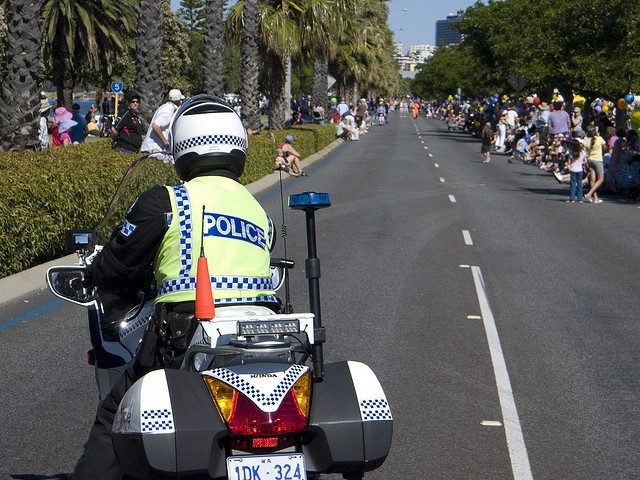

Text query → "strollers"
[378,112,386,126]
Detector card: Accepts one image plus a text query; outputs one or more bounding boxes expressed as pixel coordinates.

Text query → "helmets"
[167,94,249,178]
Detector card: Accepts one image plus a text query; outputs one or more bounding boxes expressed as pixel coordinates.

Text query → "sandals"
[300,172,308,176]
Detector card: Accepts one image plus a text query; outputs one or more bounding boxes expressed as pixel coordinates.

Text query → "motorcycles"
[45,151,393,479]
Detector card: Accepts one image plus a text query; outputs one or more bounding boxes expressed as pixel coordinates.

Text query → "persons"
[71,102,88,142]
[481,122,491,163]
[86,112,99,131]
[613,126,640,197]
[505,103,518,133]
[72,94,281,480]
[549,102,571,136]
[412,100,419,118]
[353,119,375,142]
[337,119,354,142]
[141,90,186,153]
[426,94,483,135]
[111,92,142,153]
[278,136,310,177]
[275,149,300,177]
[518,97,549,135]
[584,127,605,204]
[58,119,80,146]
[572,106,582,136]
[53,107,74,148]
[419,96,426,113]
[290,94,312,113]
[531,136,567,183]
[495,109,505,150]
[40,100,53,148]
[567,139,587,204]
[507,134,531,164]
[377,96,413,123]
[336,98,377,119]
[313,101,325,125]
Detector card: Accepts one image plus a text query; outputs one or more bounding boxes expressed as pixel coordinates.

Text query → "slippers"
[594,199,603,204]
[584,194,593,202]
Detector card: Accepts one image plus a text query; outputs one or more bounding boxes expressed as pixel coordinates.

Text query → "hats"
[538,102,549,110]
[39,99,52,114]
[127,91,140,100]
[53,107,72,124]
[58,117,78,134]
[285,135,294,142]
[168,89,185,101]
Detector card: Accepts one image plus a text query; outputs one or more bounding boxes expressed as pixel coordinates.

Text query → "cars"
[72,99,114,129]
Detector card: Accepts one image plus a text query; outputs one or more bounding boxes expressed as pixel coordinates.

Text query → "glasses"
[129,100,140,103]
